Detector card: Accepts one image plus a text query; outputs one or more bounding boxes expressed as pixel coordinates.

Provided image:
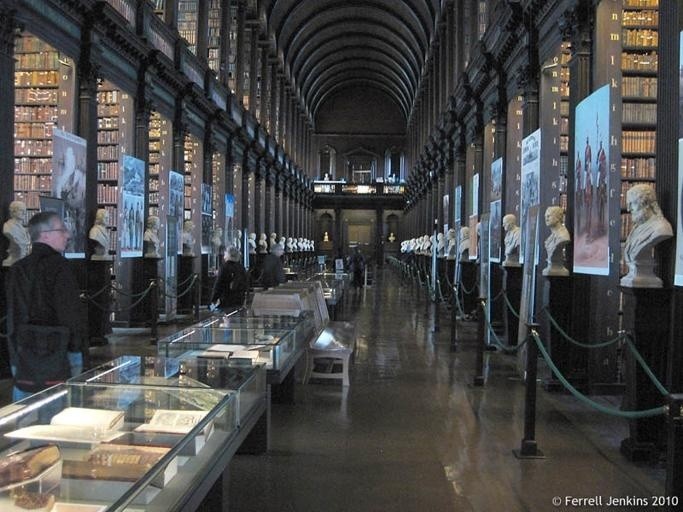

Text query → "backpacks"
[223,263,247,306]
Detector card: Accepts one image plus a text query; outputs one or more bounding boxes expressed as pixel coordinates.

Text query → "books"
[0,407,214,505]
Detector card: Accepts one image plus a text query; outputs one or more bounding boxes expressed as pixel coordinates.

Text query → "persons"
[208,244,288,316]
[0,198,33,268]
[345,247,365,289]
[86,209,314,262]
[620,182,673,287]
[501,213,521,267]
[540,205,569,277]
[400,223,480,259]
[5,212,89,405]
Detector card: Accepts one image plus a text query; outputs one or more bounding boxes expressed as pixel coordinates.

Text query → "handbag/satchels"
[13,325,71,393]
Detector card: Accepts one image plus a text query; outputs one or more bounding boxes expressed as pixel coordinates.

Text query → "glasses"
[40,226,67,235]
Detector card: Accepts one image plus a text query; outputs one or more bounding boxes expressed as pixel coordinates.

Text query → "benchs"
[308,320,356,387]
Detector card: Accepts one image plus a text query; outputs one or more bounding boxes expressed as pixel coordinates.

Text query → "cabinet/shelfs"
[0,305,316,512]
[13,0,280,256]
[619,0,659,242]
[561,41,570,218]
[305,277,346,320]
